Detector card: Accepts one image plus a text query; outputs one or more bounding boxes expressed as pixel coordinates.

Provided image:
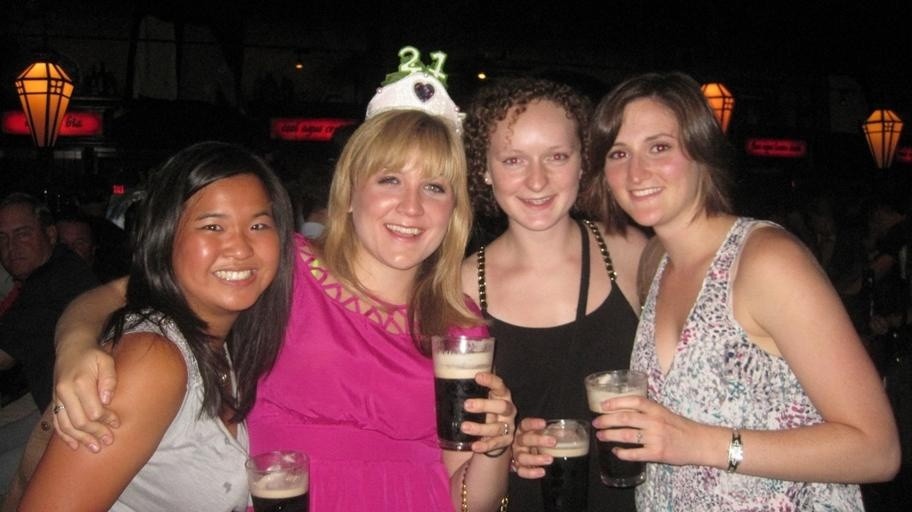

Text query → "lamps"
[862,103,907,178]
[14,57,74,168]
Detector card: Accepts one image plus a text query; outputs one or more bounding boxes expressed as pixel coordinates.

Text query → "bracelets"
[460,459,509,512]
[479,446,508,458]
[725,426,746,476]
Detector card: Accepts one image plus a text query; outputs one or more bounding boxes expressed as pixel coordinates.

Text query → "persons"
[576,70,904,511]
[435,63,652,512]
[1,177,144,510]
[51,112,500,512]
[10,139,296,512]
[751,163,910,511]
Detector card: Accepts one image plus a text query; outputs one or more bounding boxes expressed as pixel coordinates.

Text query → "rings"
[502,421,511,435]
[637,429,643,443]
[510,456,518,474]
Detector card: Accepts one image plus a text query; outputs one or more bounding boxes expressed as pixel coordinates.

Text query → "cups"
[429,334,498,453]
[243,449,312,512]
[583,369,651,489]
[534,417,592,512]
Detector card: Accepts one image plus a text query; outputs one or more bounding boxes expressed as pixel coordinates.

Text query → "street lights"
[861,110,904,182]
[15,53,75,210]
